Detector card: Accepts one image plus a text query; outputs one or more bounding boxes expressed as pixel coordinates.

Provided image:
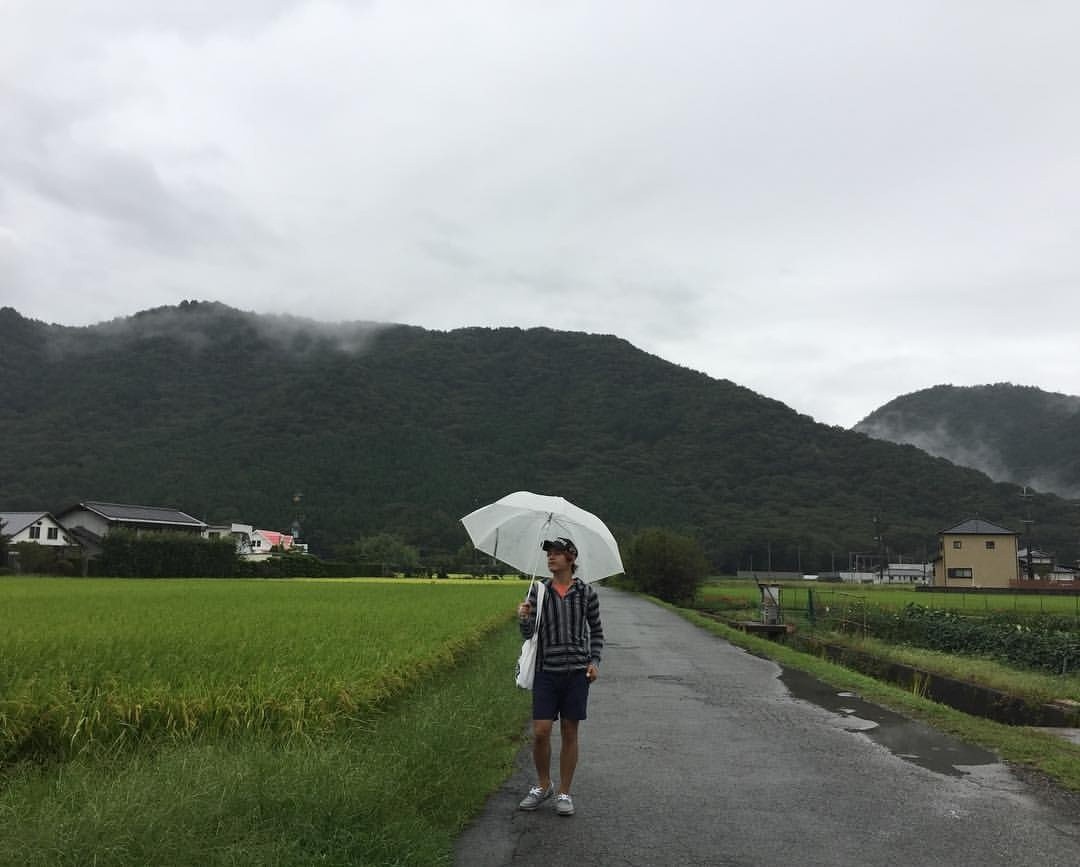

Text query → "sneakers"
[519,780,554,810]
[554,792,575,815]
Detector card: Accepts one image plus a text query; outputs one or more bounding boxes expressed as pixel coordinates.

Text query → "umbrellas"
[460,490,625,604]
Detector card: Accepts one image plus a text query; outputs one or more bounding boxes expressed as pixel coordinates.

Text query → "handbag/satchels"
[514,632,539,690]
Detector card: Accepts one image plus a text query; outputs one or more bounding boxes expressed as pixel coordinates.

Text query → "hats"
[540,536,579,556]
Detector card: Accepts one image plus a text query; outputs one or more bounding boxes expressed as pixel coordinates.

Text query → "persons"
[518,537,603,815]
